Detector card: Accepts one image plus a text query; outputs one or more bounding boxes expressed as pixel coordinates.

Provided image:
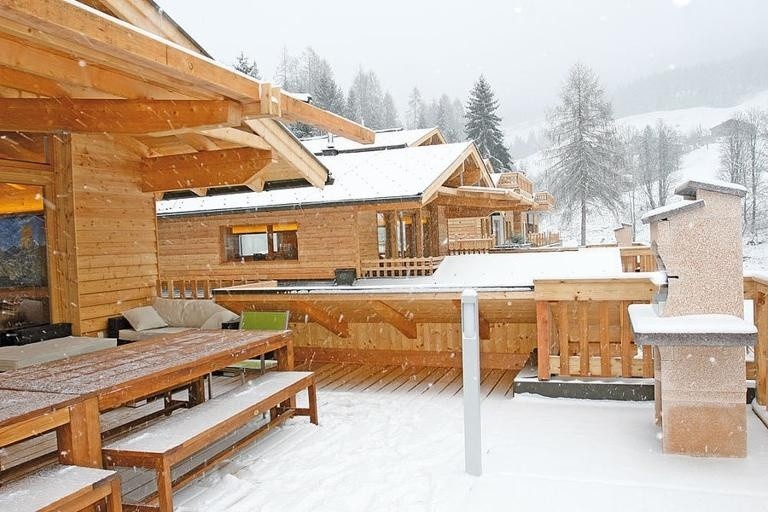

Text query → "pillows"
[202,310,238,330]
[120,307,169,333]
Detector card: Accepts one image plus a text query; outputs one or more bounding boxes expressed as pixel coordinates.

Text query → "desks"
[1,388,87,486]
[1,337,117,373]
[1,330,293,469]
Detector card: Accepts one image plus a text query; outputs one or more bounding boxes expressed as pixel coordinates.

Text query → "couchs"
[107,294,236,338]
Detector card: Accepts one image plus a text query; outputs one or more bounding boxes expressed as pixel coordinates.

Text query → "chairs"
[228,310,291,376]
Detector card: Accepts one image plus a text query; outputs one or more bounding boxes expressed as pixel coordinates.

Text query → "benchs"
[100,369,316,512]
[2,465,122,510]
